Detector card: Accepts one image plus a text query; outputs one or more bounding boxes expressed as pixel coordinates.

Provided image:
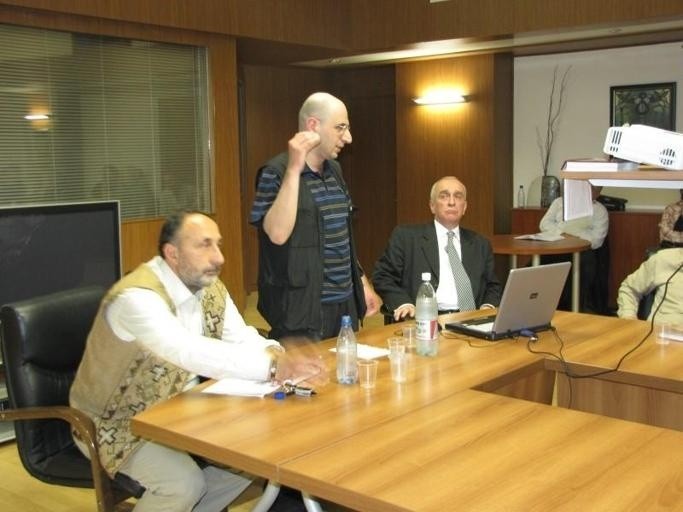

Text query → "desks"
[544,319,683,432]
[277,388,683,512]
[129,306,636,512]
[487,232,592,313]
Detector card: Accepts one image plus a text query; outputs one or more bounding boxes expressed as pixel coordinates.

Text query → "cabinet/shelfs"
[511,205,665,313]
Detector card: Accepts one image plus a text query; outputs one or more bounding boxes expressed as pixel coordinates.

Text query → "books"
[201,366,321,398]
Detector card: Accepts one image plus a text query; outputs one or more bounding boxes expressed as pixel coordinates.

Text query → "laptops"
[445,261,572,343]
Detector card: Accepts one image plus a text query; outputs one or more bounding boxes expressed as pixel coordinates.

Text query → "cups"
[388,337,405,365]
[391,353,408,382]
[655,321,670,345]
[403,325,417,348]
[357,359,377,389]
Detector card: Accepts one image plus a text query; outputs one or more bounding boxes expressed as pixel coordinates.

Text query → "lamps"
[24,114,51,121]
[408,93,470,106]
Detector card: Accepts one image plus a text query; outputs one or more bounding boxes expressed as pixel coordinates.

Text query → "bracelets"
[270,358,278,383]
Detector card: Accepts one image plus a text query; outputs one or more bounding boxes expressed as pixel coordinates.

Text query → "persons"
[660,188,683,247]
[248,91,383,343]
[370,176,504,324]
[539,183,610,313]
[616,248,683,324]
[69,212,285,512]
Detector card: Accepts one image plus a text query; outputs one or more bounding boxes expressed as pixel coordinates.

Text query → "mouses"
[438,323,443,332]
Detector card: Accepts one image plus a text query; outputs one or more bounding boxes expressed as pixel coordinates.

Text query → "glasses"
[315,117,353,135]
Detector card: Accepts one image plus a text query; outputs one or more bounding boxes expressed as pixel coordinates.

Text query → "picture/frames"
[609,81,677,132]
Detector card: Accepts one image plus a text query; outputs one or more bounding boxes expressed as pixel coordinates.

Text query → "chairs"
[1,283,229,512]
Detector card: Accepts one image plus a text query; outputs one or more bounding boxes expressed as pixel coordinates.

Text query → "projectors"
[601,123,683,173]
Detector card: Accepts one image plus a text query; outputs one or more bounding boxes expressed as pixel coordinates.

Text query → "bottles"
[336,315,358,385]
[414,273,439,356]
[518,185,525,207]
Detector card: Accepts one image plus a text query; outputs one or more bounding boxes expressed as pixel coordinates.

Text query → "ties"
[444,232,476,312]
